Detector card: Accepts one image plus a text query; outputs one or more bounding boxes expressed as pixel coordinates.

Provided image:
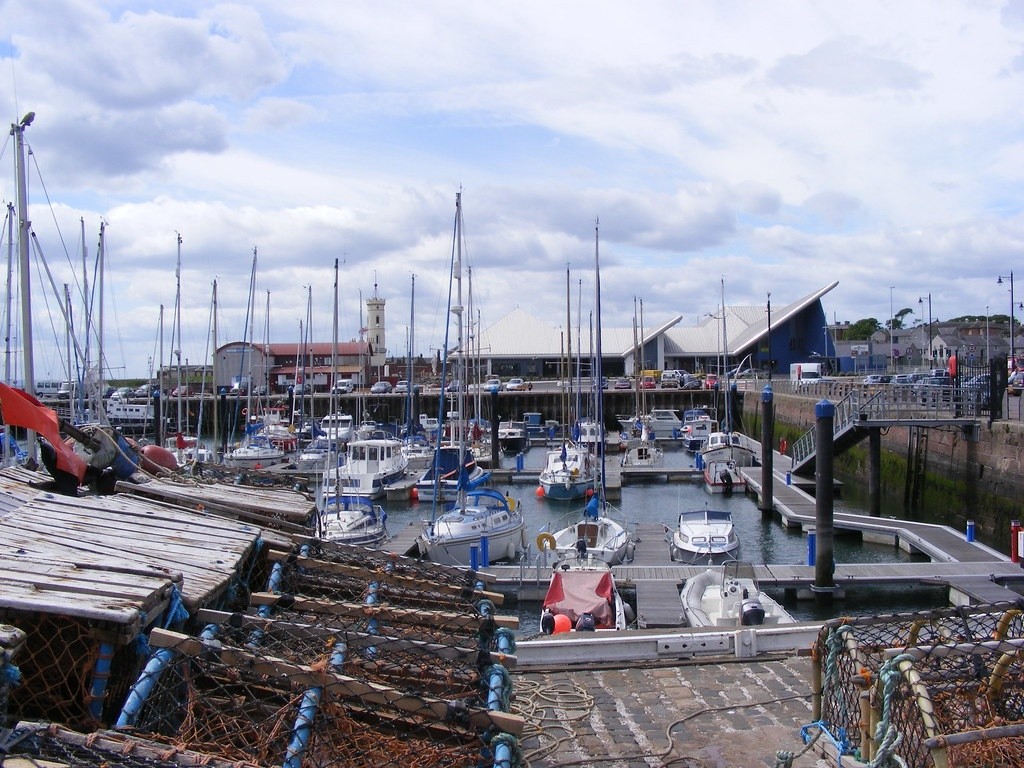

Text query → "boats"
[704,459,748,496]
[321,439,412,503]
[679,533,799,628]
[538,538,638,635]
[658,501,742,565]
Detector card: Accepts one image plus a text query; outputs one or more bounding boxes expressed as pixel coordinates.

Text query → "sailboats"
[313,259,388,549]
[0,108,751,483]
[413,187,533,567]
[415,264,491,504]
[542,216,644,566]
[538,260,599,501]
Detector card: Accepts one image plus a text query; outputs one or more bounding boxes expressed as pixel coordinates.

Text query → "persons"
[139,435,149,447]
[1008,364,1018,384]
[528,382,533,393]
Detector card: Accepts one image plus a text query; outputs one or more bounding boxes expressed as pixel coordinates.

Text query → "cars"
[483,379,504,392]
[172,385,195,397]
[370,381,393,394]
[252,385,272,396]
[446,380,467,393]
[293,383,315,395]
[394,380,412,393]
[737,368,769,379]
[862,368,994,412]
[1008,369,1024,394]
[639,376,657,389]
[110,387,135,399]
[614,378,632,389]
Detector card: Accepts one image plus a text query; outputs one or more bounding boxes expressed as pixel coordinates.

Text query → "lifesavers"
[242,407,248,415]
[288,426,295,434]
[536,532,556,551]
[498,496,514,511]
[236,442,240,449]
[573,468,579,475]
[780,439,786,454]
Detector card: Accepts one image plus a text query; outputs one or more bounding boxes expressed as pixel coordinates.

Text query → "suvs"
[134,384,160,398]
[229,381,256,396]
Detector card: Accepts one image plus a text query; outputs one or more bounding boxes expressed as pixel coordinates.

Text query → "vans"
[592,376,610,390]
[705,374,718,389]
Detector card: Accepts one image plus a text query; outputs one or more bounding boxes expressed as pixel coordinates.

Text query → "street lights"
[917,293,933,370]
[890,286,897,371]
[985,305,989,365]
[996,269,1015,372]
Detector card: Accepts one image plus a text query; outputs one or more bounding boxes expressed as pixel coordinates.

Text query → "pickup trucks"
[505,378,532,391]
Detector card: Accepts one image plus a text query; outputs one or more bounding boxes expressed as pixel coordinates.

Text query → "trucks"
[790,362,823,395]
[331,379,355,394]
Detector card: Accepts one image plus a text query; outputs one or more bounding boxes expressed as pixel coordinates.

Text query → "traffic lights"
[946,349,949,357]
[933,350,937,357]
